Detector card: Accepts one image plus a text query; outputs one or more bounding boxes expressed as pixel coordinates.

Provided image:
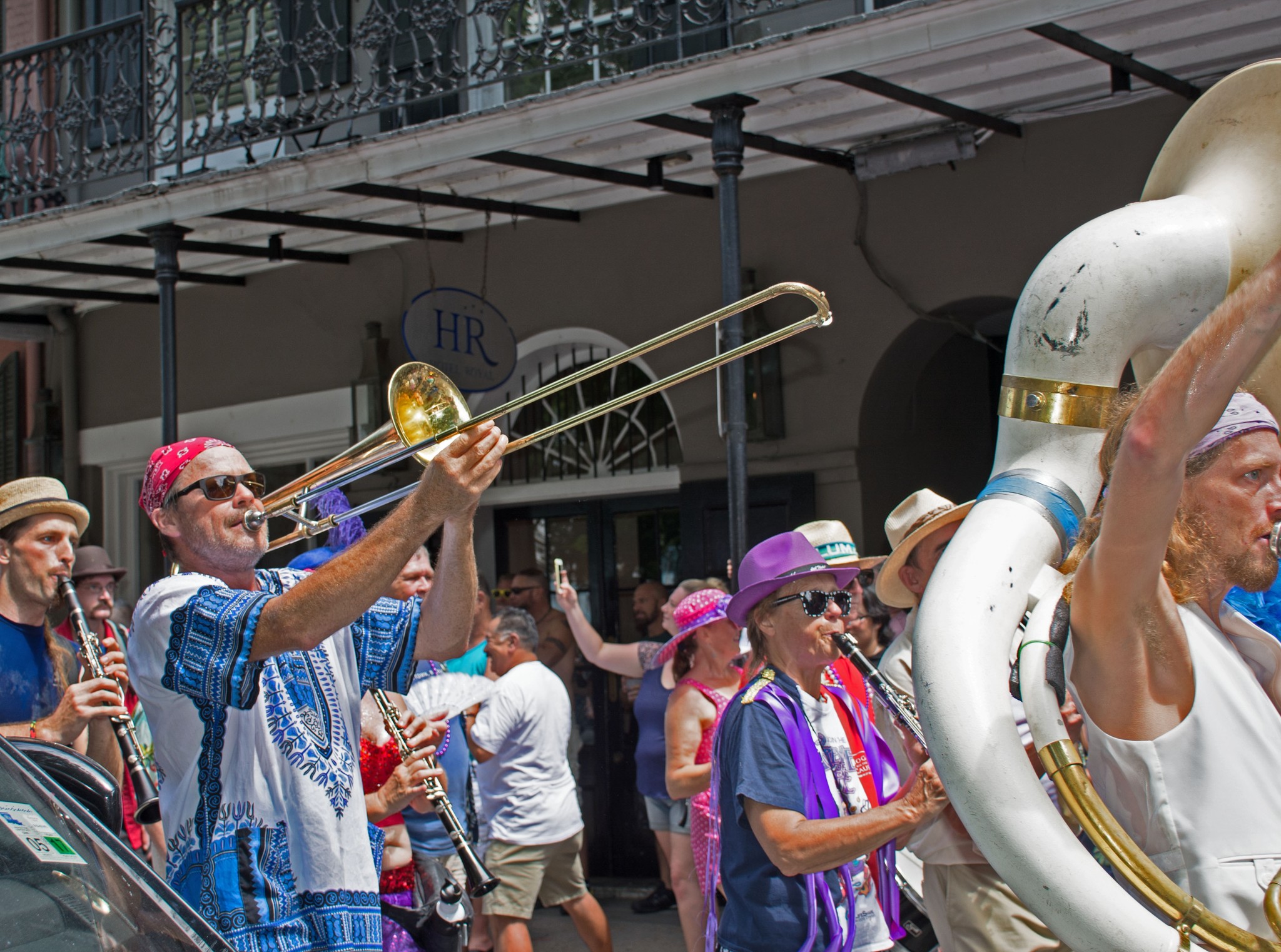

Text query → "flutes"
[373,686,500,899]
[56,576,161,824]
[830,631,932,765]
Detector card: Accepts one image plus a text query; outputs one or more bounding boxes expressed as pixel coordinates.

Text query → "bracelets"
[464,714,475,717]
[30,720,36,738]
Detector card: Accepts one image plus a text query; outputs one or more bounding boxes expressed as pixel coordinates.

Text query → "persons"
[709,533,950,952]
[53,544,166,866]
[794,520,908,724]
[287,532,615,951]
[878,487,1089,952]
[1,477,127,797]
[127,419,508,952]
[650,588,745,897]
[1068,248,1281,945]
[552,559,732,952]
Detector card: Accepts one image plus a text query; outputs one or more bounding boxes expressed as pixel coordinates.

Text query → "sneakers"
[633,880,676,913]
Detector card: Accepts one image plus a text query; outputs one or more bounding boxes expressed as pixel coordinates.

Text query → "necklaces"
[428,659,450,756]
[824,664,868,711]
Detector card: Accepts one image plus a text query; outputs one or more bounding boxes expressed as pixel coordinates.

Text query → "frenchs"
[910,56,1281,952]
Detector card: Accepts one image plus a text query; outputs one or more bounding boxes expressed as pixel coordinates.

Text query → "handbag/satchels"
[377,851,475,952]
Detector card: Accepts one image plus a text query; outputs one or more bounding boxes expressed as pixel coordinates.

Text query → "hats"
[793,521,887,569]
[650,589,736,669]
[72,546,127,582]
[874,489,977,609]
[726,531,861,626]
[0,477,90,539]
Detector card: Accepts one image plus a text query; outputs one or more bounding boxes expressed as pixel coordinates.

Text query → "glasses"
[771,590,852,617]
[164,472,267,507]
[491,589,512,600]
[77,583,120,593]
[510,586,542,595]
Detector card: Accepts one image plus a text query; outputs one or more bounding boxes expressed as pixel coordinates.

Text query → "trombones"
[244,282,831,554]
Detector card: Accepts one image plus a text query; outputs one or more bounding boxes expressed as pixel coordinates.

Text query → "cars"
[0,729,241,952]
